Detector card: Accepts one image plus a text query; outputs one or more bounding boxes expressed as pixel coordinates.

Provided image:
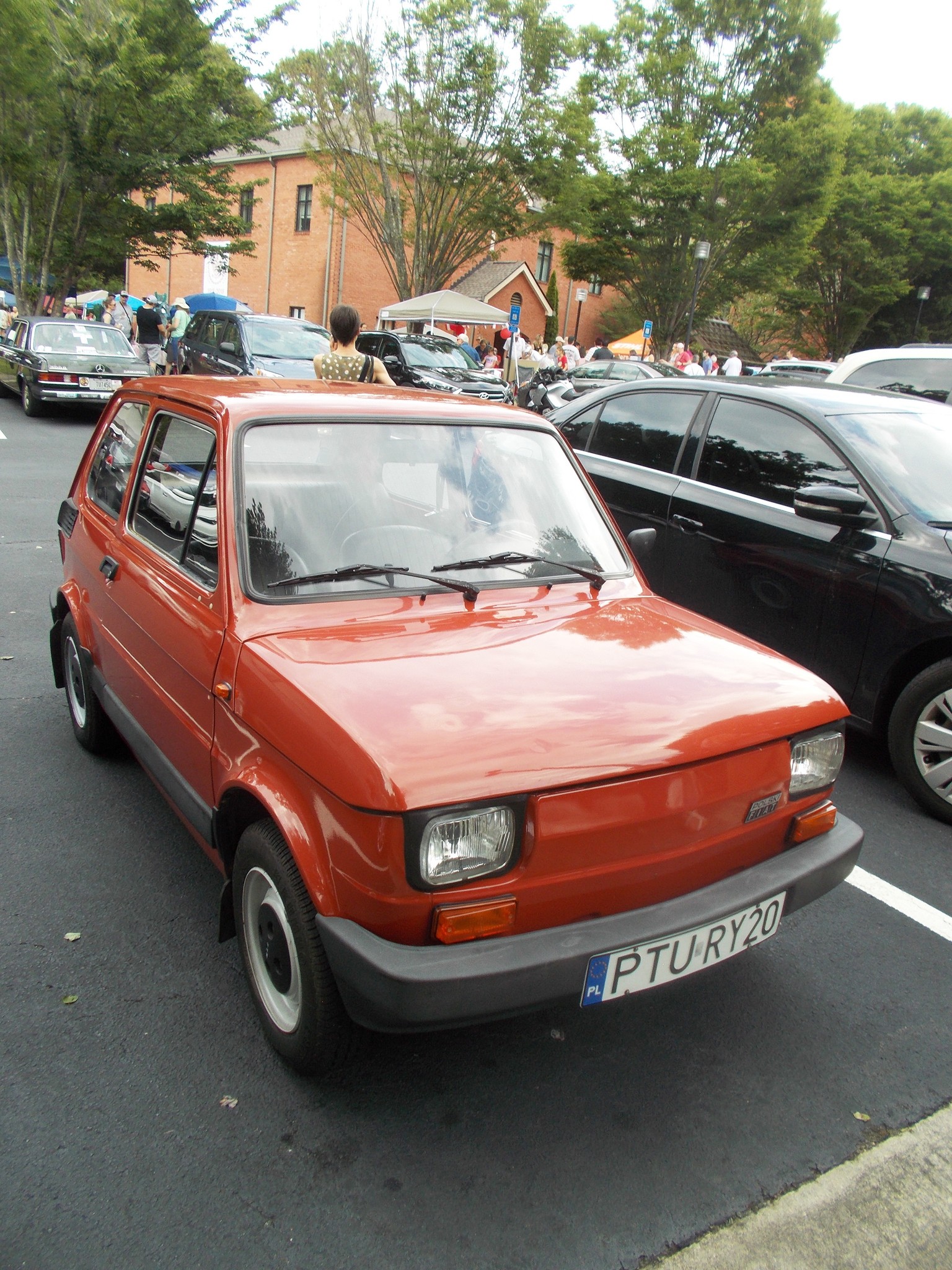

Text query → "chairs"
[512,366,535,396]
[341,524,448,585]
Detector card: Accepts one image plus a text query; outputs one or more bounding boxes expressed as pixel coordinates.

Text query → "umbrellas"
[0,289,156,322]
[170,292,254,314]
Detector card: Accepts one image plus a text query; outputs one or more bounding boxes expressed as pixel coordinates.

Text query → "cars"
[0,316,155,418]
[563,358,691,392]
[437,382,951,828]
[49,374,866,1079]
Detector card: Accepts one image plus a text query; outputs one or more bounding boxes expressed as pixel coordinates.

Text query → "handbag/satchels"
[164,331,171,353]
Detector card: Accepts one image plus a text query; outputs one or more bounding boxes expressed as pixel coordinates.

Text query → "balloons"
[500,324,521,339]
[449,324,469,343]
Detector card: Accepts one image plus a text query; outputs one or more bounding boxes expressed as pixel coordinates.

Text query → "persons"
[65,304,76,319]
[475,335,501,369]
[658,341,742,377]
[86,311,95,321]
[629,350,641,362]
[0,303,19,336]
[360,323,368,332]
[816,353,843,374]
[643,345,656,363]
[313,304,397,387]
[503,333,613,372]
[101,291,193,376]
[770,349,798,363]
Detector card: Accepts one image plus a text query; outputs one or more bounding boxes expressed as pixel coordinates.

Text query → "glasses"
[121,295,127,298]
[476,339,480,341]
[110,304,116,306]
[457,339,460,341]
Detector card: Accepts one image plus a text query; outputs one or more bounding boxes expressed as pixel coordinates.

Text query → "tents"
[607,328,654,362]
[377,289,519,389]
[0,255,78,320]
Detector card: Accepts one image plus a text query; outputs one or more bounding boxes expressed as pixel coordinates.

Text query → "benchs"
[245,477,392,558]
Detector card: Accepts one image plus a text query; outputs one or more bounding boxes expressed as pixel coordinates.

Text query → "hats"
[121,290,128,296]
[3,304,9,309]
[109,293,116,298]
[171,297,190,309]
[147,295,157,303]
[556,336,566,343]
[65,303,74,308]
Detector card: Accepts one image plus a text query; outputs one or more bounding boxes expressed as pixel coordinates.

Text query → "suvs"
[355,329,514,405]
[175,309,332,381]
[740,343,952,407]
